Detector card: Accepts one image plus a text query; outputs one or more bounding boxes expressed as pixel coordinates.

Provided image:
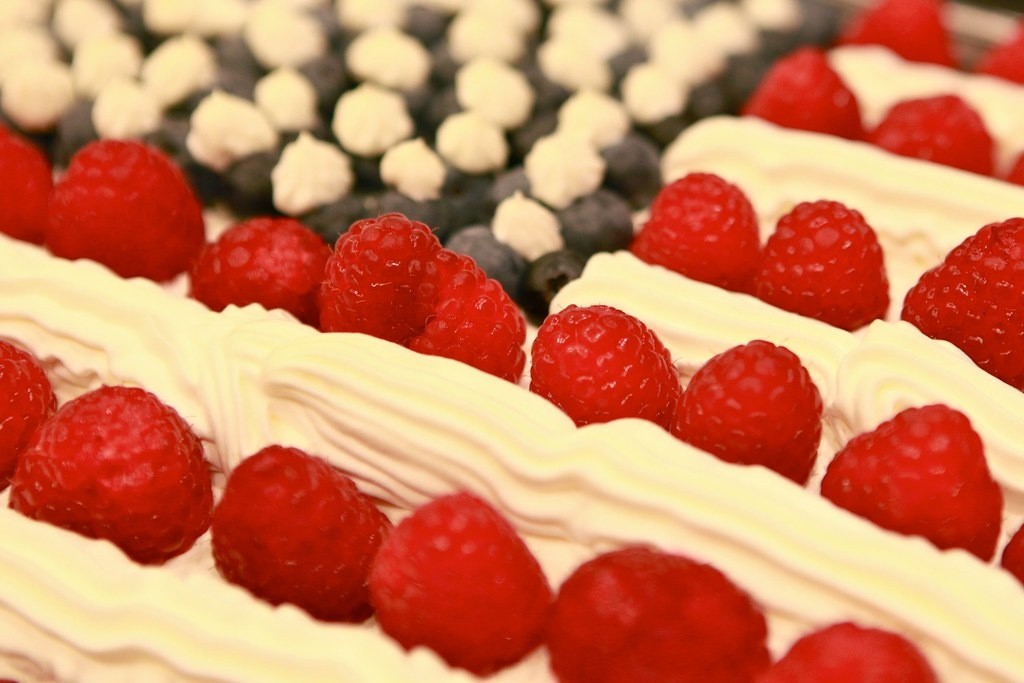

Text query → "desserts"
[0,0,1024,683]
